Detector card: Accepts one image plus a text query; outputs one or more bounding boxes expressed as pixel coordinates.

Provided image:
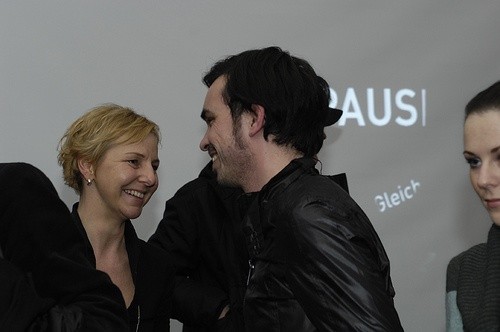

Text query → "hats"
[325,108,343,126]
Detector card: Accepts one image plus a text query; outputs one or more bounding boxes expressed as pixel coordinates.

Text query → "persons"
[146,80,349,332]
[199,45,405,332]
[1,104,171,331]
[444,78,500,332]
